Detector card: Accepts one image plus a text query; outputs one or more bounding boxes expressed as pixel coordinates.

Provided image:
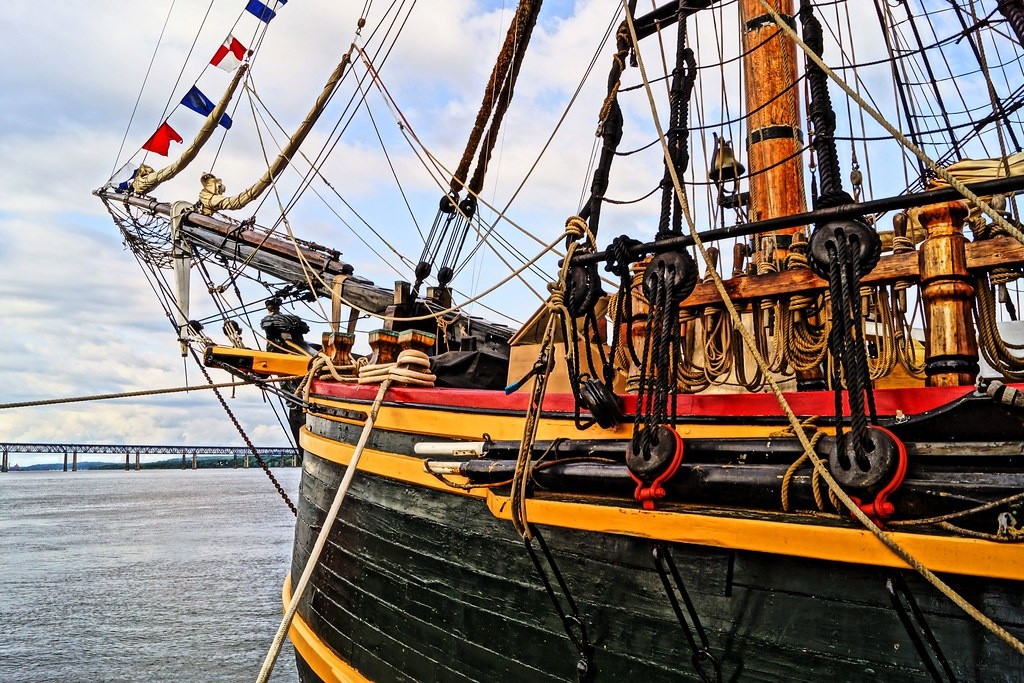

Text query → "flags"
[141,122,183,157]
[246,0,288,23]
[180,85,233,130]
[210,34,254,73]
[110,163,139,195]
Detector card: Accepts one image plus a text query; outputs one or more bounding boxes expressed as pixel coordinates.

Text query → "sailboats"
[90,0,1023,683]
